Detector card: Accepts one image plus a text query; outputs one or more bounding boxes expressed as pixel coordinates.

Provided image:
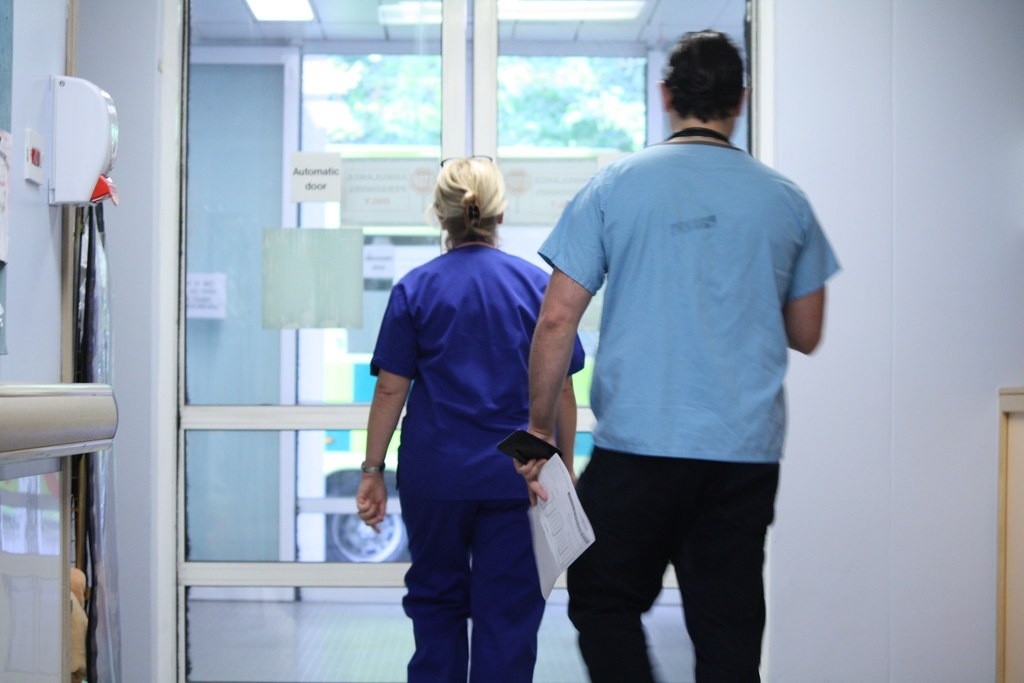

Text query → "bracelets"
[361,460,385,474]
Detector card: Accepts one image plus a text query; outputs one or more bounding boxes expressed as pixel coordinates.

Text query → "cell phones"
[497,430,562,464]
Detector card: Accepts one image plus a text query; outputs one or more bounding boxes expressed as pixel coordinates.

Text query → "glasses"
[441,155,493,167]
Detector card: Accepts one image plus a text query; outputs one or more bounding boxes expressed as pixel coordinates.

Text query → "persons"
[356,155,585,683]
[512,29,843,683]
